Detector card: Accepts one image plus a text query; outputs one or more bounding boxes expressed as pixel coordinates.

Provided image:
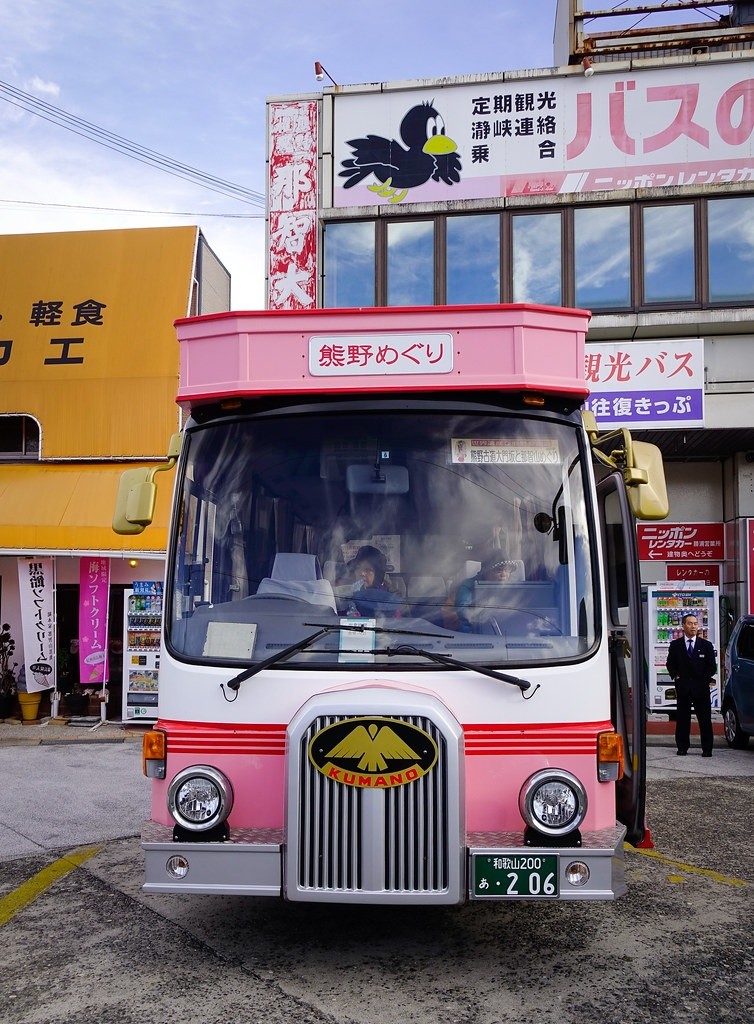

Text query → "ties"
[687,639,694,658]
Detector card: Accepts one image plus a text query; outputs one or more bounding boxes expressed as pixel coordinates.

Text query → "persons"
[454,549,518,633]
[665,614,717,757]
[340,546,410,618]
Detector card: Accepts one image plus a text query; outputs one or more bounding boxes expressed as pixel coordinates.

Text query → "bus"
[107,297,672,910]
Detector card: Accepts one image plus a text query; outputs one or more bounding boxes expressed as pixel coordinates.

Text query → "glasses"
[493,566,513,574]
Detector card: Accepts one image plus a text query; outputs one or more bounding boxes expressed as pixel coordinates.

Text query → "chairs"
[257,553,525,628]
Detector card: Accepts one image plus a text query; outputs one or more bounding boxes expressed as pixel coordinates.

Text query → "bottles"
[130,618,161,626]
[658,612,708,626]
[350,579,365,592]
[347,601,360,617]
[128,633,160,646]
[657,629,684,640]
[129,596,162,611]
[658,595,703,607]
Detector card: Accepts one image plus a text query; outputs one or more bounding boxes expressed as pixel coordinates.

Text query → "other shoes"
[702,751,712,757]
[677,749,688,756]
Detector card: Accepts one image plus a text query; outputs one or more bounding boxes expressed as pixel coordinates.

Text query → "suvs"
[719,614,754,750]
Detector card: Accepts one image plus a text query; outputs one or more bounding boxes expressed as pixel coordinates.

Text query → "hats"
[347,545,394,573]
[481,549,517,574]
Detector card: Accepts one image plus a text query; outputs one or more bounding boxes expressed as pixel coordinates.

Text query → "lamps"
[128,559,141,569]
[315,62,337,86]
[583,57,594,78]
